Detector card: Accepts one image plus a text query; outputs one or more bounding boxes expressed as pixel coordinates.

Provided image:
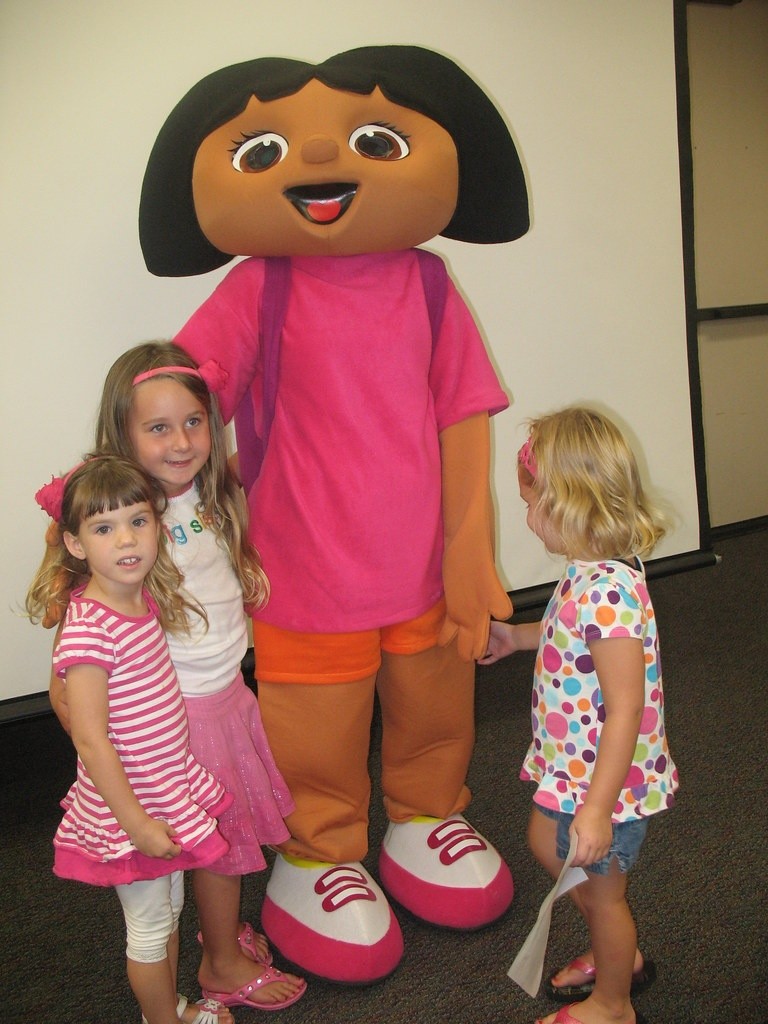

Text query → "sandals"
[141,994,236,1024]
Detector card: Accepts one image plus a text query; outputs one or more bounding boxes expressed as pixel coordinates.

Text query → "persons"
[38,45,532,985]
[53,455,236,1024]
[478,410,680,1024]
[26,343,308,1011]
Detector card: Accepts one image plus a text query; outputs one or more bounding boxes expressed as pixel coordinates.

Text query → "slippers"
[202,969,307,1010]
[544,954,657,1000]
[527,1000,646,1024]
[197,922,276,965]
